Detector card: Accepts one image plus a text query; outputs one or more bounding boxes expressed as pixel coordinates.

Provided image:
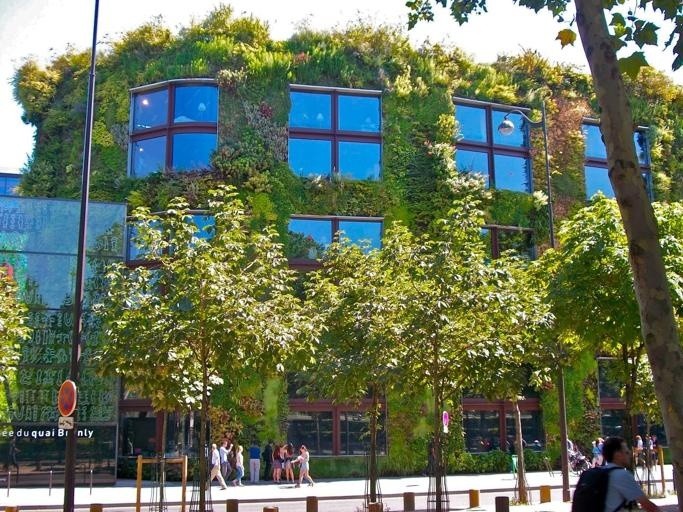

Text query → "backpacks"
[571,466,626,511]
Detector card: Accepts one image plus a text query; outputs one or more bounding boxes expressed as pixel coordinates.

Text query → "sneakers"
[272,480,315,488]
[219,481,245,491]
[250,480,261,484]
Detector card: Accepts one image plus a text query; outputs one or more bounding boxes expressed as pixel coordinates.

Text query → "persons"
[560,432,658,472]
[248,440,260,484]
[282,441,295,483]
[204,443,227,491]
[270,444,283,483]
[599,435,662,511]
[126,438,133,456]
[290,445,313,490]
[219,440,234,483]
[2,435,20,470]
[231,445,245,487]
[263,439,272,481]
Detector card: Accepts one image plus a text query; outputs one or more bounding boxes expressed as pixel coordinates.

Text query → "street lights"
[498,86,571,502]
